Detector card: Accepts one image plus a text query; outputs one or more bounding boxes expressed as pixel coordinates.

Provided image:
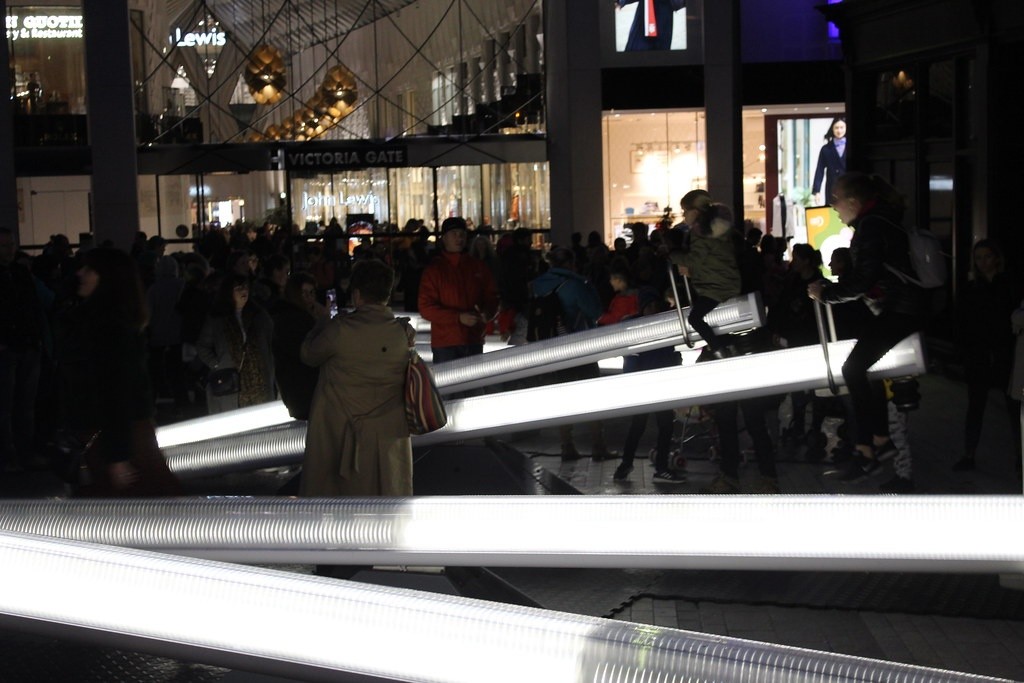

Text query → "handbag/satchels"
[210,370,240,396]
[405,349,447,435]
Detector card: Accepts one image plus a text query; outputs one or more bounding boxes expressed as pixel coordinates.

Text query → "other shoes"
[755,468,780,481]
[723,471,745,487]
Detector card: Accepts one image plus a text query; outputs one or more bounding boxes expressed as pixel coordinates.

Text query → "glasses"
[302,288,318,297]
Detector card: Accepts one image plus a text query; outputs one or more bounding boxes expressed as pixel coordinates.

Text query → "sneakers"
[874,439,898,461]
[838,450,882,482]
[651,469,688,484]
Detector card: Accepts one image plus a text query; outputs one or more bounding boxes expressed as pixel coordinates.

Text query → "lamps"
[244,0,358,143]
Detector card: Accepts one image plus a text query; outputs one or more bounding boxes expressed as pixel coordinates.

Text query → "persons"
[811,117,846,207]
[0,162,1024,580]
[27,73,41,106]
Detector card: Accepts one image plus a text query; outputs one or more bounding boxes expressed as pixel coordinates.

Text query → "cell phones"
[326,289,338,315]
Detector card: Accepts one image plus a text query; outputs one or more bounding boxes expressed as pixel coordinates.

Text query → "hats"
[442,217,467,233]
